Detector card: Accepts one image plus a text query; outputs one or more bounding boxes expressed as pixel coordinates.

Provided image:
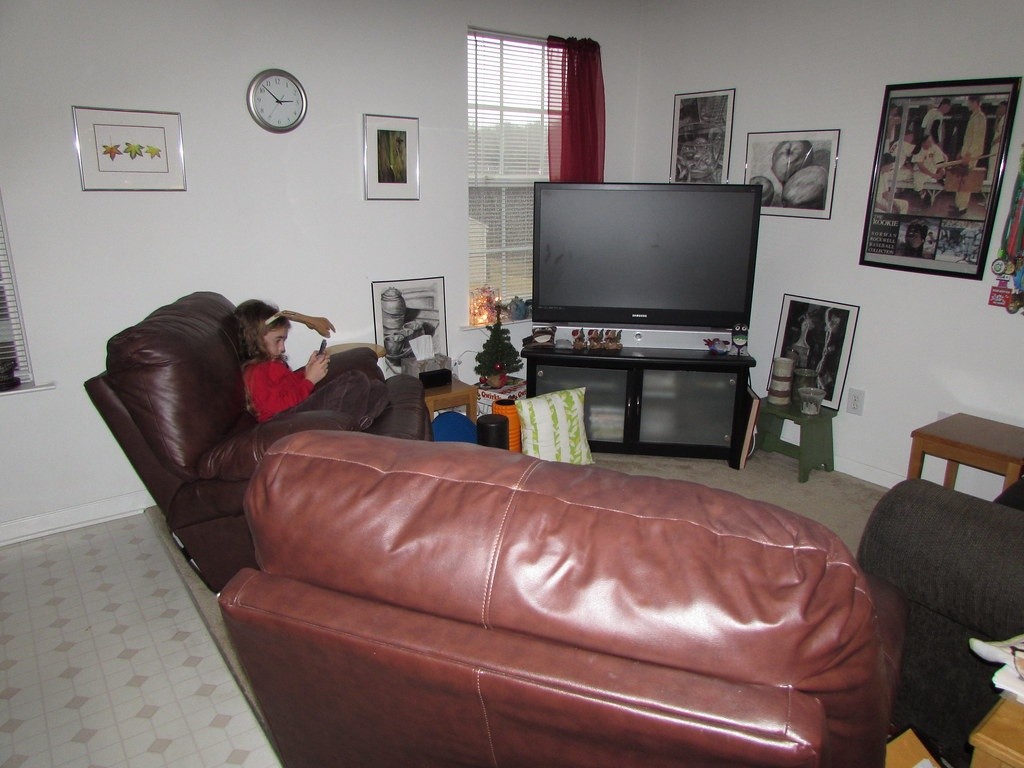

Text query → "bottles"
[792,369,818,407]
[510,296,525,320]
[525,299,532,319]
[767,357,794,406]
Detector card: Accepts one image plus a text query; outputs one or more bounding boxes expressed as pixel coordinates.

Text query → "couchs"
[857,479,1024,768]
[216,427,899,768]
[83,290,433,598]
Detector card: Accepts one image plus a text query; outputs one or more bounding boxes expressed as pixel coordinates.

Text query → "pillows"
[514,387,595,465]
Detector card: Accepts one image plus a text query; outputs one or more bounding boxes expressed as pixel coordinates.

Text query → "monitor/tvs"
[531,182,763,330]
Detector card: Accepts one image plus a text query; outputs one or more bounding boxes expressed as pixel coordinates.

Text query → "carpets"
[144,451,892,745]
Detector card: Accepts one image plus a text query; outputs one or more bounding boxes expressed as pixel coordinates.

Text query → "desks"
[747,397,838,482]
[969,697,1024,768]
[473,376,526,415]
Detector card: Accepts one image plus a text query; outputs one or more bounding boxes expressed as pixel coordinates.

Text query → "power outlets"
[846,387,866,415]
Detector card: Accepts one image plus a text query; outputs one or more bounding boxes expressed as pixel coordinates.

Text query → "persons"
[874,94,1009,265]
[231,299,391,428]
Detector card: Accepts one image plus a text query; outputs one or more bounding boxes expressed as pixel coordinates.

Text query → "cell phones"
[318,340,327,356]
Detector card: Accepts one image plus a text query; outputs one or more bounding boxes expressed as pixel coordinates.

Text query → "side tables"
[425,379,477,422]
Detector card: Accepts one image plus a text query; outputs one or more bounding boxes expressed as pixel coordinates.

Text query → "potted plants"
[477,307,523,389]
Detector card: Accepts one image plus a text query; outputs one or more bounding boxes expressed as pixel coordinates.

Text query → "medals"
[991,253,1024,275]
[1005,292,1024,318]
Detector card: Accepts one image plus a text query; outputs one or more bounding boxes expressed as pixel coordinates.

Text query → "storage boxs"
[419,368,453,387]
[884,728,942,768]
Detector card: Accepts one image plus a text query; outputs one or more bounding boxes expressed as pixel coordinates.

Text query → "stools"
[906,413,1024,493]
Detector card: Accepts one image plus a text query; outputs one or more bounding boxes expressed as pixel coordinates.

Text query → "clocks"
[246,69,308,134]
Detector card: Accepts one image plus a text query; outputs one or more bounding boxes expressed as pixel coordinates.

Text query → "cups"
[798,388,827,415]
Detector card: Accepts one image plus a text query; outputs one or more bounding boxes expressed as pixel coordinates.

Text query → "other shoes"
[948,208,967,218]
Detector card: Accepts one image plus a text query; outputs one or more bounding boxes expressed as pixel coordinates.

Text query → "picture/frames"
[72,105,187,191]
[743,129,840,220]
[371,276,448,379]
[858,76,1023,282]
[362,113,420,201]
[669,88,736,183]
[765,293,860,411]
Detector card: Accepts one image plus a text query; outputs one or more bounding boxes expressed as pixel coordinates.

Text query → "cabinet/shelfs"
[519,346,757,470]
